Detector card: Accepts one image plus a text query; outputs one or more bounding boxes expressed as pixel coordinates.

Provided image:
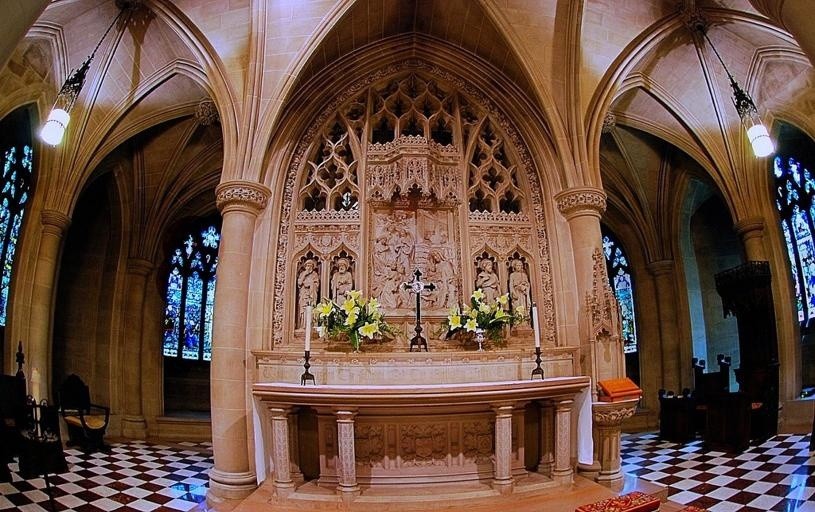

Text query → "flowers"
[315,290,399,351]
[444,289,525,343]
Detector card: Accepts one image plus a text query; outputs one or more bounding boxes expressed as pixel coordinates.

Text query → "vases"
[472,330,487,352]
[350,337,364,353]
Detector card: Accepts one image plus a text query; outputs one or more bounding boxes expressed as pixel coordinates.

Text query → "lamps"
[38,11,122,146]
[701,31,774,158]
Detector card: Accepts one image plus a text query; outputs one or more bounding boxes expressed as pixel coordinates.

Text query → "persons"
[298,259,319,329]
[476,259,501,307]
[510,258,533,324]
[372,223,457,309]
[330,258,353,307]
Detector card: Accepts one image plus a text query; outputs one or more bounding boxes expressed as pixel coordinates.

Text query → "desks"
[252,376,590,499]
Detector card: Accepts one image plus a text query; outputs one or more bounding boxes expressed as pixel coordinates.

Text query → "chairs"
[52,373,117,456]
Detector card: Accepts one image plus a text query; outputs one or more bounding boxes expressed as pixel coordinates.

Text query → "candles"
[533,307,541,347]
[305,306,312,350]
